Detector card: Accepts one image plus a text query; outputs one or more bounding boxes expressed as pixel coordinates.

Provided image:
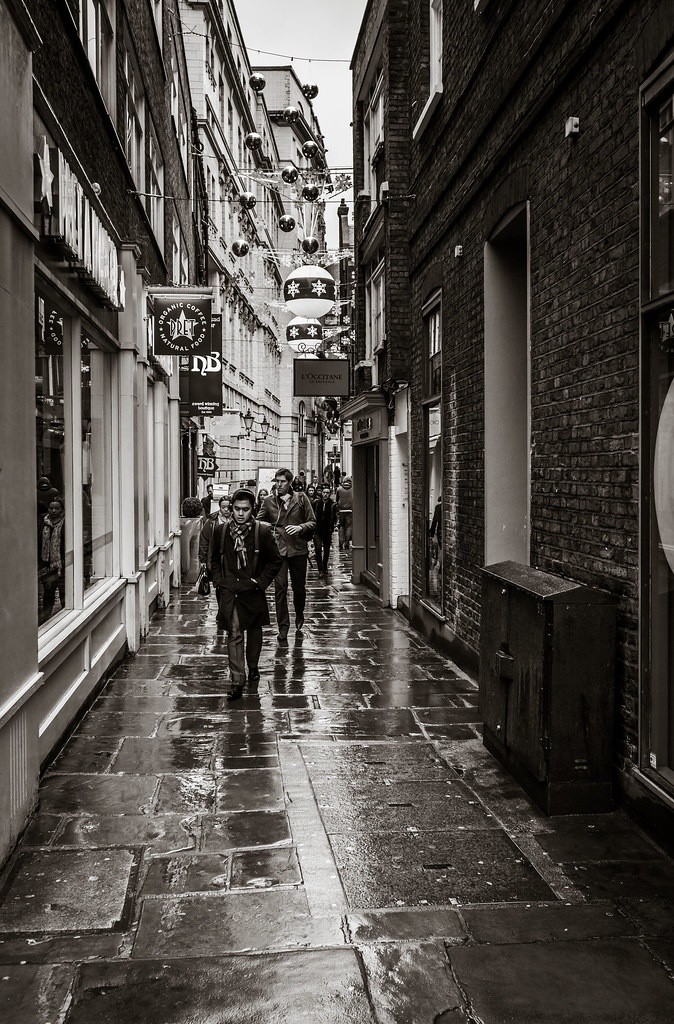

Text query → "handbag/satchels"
[198,569,210,595]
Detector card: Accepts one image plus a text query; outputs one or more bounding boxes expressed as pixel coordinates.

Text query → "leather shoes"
[296,610,304,629]
[228,684,242,696]
[277,631,287,641]
[249,666,260,681]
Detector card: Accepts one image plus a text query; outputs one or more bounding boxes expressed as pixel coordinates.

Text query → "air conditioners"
[564,117,580,137]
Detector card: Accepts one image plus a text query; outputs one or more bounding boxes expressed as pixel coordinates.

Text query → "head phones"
[228,490,257,518]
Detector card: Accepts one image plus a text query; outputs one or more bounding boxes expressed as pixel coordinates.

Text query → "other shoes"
[318,569,328,579]
[344,544,349,551]
[339,547,343,552]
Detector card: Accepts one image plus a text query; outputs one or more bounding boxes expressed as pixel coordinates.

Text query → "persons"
[311,466,352,579]
[201,465,318,698]
[37,490,91,620]
[430,496,442,549]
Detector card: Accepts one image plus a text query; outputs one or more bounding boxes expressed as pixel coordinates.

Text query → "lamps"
[232,409,255,440]
[255,415,271,442]
